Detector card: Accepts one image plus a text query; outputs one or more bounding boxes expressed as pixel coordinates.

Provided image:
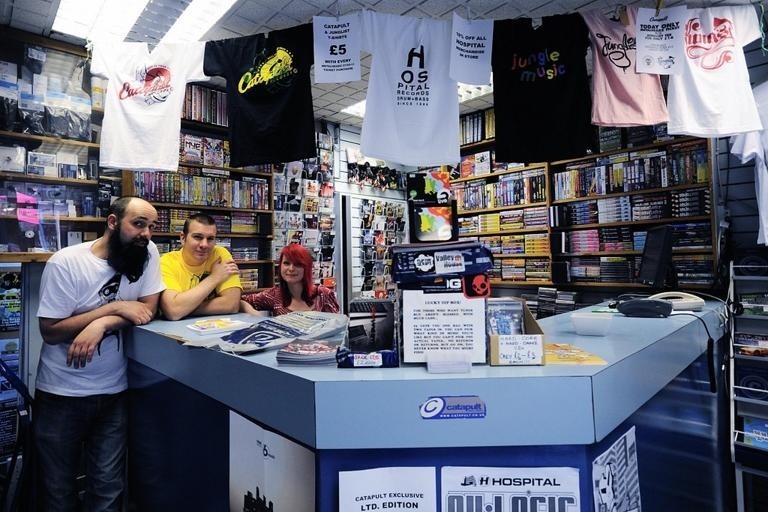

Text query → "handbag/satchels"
[181,311,349,356]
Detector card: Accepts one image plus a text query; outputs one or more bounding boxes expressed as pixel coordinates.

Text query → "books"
[542,341,608,367]
[189,317,252,337]
[459,110,494,145]
[737,291,768,316]
[271,135,409,296]
[134,133,269,291]
[180,83,228,127]
[734,332,768,357]
[741,414,768,450]
[551,145,715,284]
[522,287,594,318]
[455,169,551,281]
[274,337,342,367]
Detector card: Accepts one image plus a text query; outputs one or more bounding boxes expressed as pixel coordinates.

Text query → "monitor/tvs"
[637,224,676,291]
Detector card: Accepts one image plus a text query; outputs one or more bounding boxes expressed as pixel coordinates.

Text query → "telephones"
[644,291,705,312]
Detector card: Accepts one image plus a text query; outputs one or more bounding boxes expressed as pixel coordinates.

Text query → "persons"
[157,213,244,322]
[239,242,341,319]
[29,194,168,511]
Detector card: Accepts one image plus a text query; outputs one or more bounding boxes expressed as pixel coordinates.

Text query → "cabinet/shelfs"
[1,24,122,265]
[725,261,766,512]
[446,161,552,287]
[129,81,275,299]
[551,130,722,293]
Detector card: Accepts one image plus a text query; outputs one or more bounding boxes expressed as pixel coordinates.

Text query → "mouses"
[608,300,621,308]
